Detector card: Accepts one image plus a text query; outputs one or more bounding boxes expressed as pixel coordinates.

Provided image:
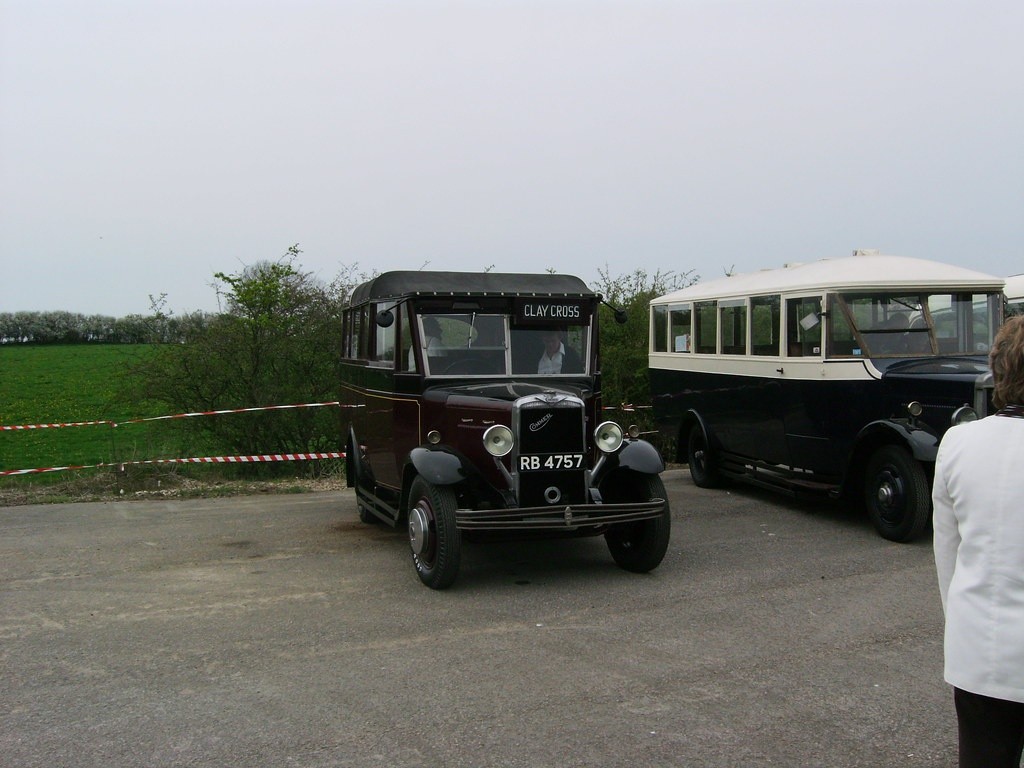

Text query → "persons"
[933,314,1024,768]
[530,326,585,374]
[407,316,444,371]
[847,313,909,356]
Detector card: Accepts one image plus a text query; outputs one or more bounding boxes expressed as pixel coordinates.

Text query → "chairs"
[789,337,957,357]
[428,349,515,373]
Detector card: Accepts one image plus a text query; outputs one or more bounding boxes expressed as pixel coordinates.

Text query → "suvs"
[336,268,671,592]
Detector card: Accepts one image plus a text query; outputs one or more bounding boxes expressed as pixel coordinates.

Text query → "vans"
[900,273,1023,360]
[646,250,1006,541]
